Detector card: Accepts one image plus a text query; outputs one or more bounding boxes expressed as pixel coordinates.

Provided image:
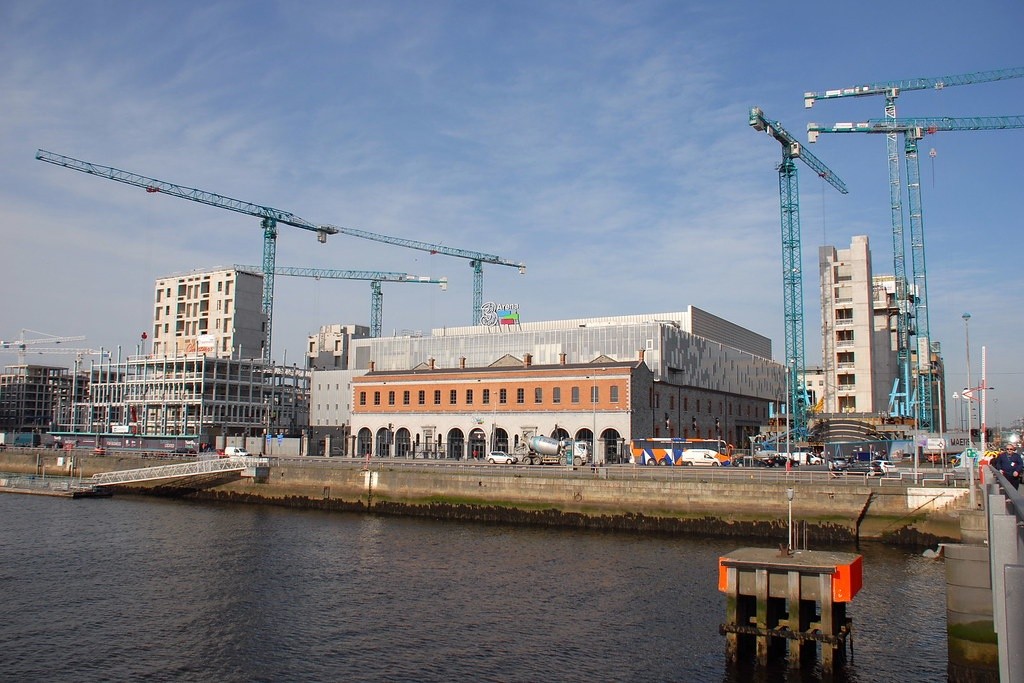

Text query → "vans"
[12,433,41,448]
[0,432,7,449]
[792,451,823,466]
[224,446,253,458]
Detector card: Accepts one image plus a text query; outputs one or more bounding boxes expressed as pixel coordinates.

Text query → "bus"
[627,437,731,467]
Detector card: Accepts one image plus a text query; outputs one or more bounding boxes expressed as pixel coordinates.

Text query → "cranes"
[326,223,527,327]
[0,328,88,365]
[0,346,110,372]
[34,148,334,365]
[803,66,1022,435]
[748,105,849,444]
[234,263,447,337]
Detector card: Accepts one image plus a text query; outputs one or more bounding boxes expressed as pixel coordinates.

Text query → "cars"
[760,454,800,468]
[731,454,767,468]
[44,440,63,449]
[319,446,346,457]
[846,451,898,477]
[827,456,849,474]
[484,450,518,464]
[169,447,198,458]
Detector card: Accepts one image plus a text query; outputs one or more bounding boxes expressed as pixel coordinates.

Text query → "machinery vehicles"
[519,431,590,466]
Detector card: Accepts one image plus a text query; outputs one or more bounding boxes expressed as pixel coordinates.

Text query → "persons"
[990,444,1023,500]
[473,449,479,462]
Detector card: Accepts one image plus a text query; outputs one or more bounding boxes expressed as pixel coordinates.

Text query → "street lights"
[964,387,994,430]
[491,391,497,451]
[592,366,606,470]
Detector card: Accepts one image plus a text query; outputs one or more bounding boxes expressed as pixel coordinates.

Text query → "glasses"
[1007,449,1013,450]
